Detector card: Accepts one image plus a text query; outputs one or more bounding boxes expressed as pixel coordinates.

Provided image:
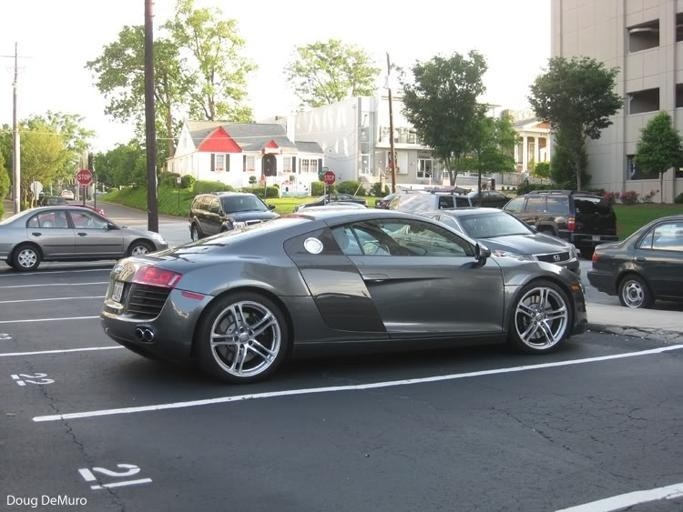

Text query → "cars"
[0,188,682,312]
[466,172,491,186]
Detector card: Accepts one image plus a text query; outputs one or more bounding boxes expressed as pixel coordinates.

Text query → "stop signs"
[322,170,335,185]
[76,170,92,185]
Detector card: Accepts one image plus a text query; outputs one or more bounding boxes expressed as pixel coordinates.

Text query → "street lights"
[381,75,396,193]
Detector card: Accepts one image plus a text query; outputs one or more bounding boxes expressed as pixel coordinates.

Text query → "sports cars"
[98,207,590,384]
